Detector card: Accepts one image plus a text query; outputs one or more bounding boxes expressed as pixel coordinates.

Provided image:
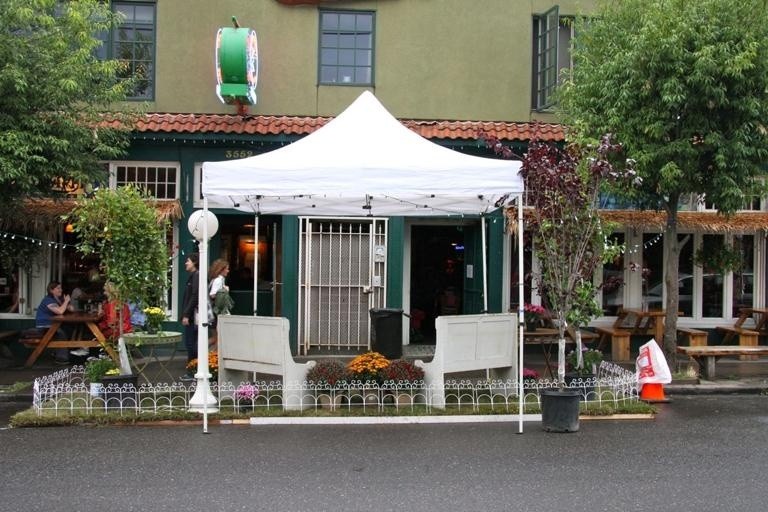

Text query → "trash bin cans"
[369,307,402,359]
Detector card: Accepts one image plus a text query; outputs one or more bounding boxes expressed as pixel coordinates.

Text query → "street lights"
[187,209,219,412]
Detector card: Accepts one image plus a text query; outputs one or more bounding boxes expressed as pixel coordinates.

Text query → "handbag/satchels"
[193,299,214,325]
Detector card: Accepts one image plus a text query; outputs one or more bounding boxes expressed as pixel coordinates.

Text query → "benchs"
[414,308,522,413]
[677,343,768,381]
[21,328,48,369]
[214,313,317,413]
[535,324,632,361]
[716,323,760,361]
[677,327,711,345]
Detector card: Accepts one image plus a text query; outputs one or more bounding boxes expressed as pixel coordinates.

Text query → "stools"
[1,328,20,361]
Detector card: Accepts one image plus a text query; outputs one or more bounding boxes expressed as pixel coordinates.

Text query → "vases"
[317,389,415,411]
[146,321,159,333]
[525,321,536,332]
[101,375,138,414]
[239,400,253,412]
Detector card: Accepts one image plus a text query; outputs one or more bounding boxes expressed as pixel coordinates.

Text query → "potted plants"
[565,276,603,400]
[85,356,117,398]
[479,118,642,432]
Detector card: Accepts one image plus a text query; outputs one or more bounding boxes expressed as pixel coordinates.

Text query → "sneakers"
[179,371,196,382]
[70,348,89,357]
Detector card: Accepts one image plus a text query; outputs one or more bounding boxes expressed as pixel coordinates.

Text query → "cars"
[603,263,753,316]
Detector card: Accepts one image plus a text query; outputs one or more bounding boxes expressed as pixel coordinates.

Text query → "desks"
[21,312,119,371]
[521,327,600,380]
[122,330,184,386]
[597,306,686,351]
[718,305,768,345]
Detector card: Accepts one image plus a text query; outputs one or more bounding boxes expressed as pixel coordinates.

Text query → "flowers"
[186,349,218,381]
[143,306,166,326]
[304,349,425,396]
[566,347,602,374]
[517,302,545,322]
[104,367,121,377]
[232,383,259,402]
[522,367,539,380]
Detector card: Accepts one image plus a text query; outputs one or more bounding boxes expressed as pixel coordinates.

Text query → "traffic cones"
[638,383,673,403]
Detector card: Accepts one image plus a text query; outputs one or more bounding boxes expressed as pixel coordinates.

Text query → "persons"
[34,280,75,366]
[207,258,231,352]
[235,266,254,291]
[70,282,131,364]
[179,251,199,382]
[123,291,145,332]
